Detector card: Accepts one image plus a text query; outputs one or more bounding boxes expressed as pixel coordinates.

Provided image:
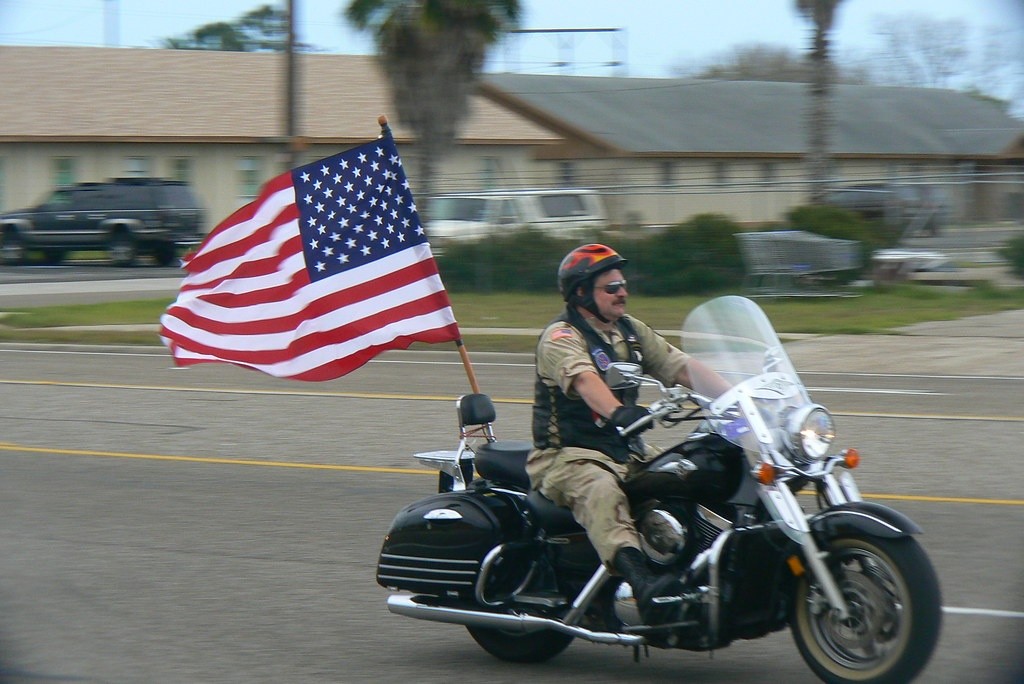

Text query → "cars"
[741,224,868,294]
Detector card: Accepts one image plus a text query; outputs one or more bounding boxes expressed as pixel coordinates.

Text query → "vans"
[418,188,613,259]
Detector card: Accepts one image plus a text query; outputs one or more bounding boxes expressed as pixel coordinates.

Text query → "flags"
[158,136,462,382]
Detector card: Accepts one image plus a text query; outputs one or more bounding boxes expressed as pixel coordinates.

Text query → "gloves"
[609,406,654,438]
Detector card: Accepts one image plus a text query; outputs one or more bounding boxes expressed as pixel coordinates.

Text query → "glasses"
[584,280,627,294]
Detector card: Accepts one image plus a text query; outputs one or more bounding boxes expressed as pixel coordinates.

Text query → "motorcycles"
[378,293,944,684]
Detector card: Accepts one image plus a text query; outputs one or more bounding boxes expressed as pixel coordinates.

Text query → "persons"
[526,243,733,624]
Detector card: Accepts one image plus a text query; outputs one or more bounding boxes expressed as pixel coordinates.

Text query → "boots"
[613,547,680,625]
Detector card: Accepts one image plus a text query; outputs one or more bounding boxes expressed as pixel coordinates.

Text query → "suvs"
[0,175,205,267]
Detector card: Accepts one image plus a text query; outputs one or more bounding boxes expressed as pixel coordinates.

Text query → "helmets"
[558,244,627,302]
